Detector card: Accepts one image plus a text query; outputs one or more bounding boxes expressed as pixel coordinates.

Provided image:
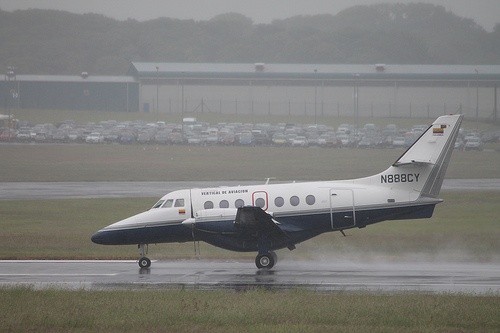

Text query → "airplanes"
[91,114,462,267]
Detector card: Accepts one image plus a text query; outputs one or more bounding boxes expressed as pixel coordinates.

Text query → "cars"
[2,115,484,149]
